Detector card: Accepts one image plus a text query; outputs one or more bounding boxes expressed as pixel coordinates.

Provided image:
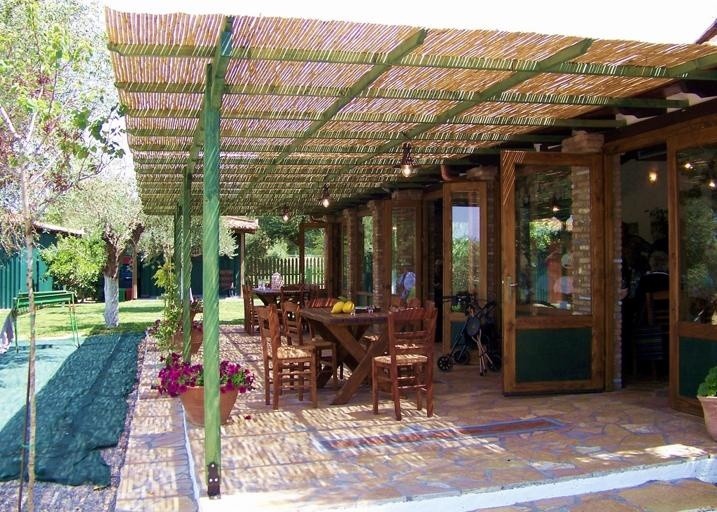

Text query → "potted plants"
[696,367,717,441]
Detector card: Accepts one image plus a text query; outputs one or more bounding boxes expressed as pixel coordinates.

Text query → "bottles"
[272,272,283,292]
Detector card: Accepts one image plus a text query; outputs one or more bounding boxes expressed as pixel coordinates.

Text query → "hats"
[561,254,574,269]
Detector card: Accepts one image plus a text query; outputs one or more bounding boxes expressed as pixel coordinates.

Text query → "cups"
[259,279,266,291]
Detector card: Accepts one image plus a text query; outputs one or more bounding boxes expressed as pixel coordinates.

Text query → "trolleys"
[437,291,502,377]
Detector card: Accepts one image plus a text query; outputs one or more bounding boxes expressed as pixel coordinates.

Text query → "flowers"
[151,352,257,397]
[192,320,203,331]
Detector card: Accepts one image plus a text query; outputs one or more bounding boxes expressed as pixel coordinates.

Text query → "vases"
[176,384,238,427]
[191,329,202,354]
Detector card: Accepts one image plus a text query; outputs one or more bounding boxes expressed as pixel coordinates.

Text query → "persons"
[395,268,415,298]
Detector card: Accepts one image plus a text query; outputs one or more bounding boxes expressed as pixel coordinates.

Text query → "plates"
[355,306,382,313]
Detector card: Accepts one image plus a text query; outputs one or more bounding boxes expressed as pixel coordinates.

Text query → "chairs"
[242,282,439,421]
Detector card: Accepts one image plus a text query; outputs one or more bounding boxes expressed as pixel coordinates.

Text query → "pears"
[330,301,344,314]
[343,301,355,314]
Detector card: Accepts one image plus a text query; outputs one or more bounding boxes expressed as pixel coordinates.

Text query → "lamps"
[322,183,330,208]
[400,143,414,178]
[283,204,290,221]
